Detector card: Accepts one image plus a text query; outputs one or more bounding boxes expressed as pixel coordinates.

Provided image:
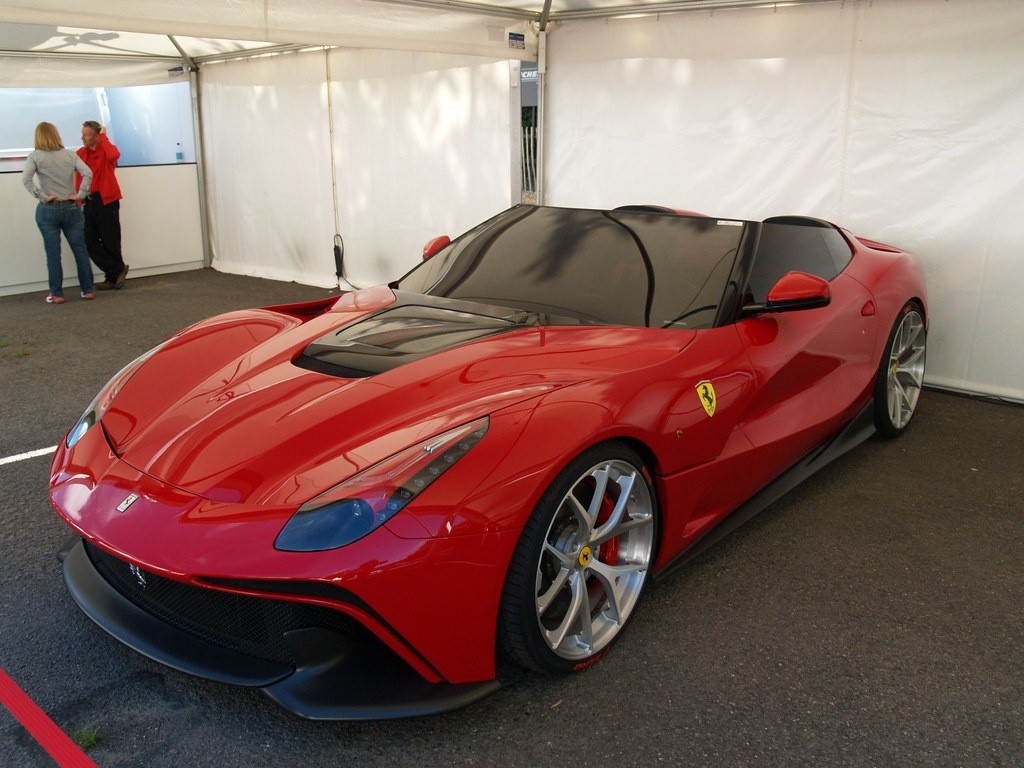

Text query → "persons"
[72,120,129,292]
[22,121,96,303]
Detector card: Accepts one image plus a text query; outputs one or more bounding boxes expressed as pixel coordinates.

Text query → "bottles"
[176,143,183,163]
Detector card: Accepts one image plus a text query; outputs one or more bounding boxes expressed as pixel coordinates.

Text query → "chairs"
[610,205,677,215]
[750,214,832,230]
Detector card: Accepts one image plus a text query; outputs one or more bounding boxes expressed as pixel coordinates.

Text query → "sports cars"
[49,203,928,720]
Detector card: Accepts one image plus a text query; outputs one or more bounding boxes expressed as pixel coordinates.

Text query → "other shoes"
[115,265,128,289]
[46,295,64,304]
[96,280,127,290]
[80,292,94,299]
